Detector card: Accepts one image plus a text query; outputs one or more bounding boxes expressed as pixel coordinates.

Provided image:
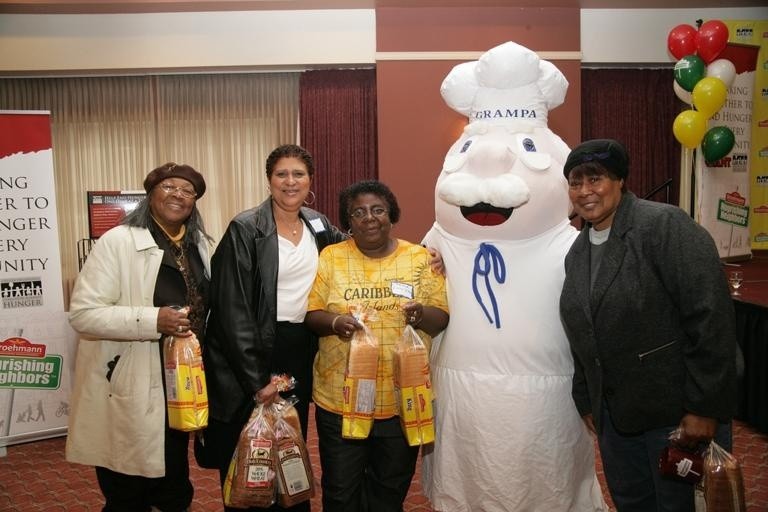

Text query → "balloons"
[668,20,737,164]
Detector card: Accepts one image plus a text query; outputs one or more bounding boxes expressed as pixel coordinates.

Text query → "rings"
[177,326,184,331]
[410,317,415,323]
[413,310,417,315]
[345,331,350,335]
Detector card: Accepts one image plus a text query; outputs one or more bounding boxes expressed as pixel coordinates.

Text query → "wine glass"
[729,269,744,296]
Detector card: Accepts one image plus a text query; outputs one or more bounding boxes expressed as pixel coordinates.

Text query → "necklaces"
[286,223,298,234]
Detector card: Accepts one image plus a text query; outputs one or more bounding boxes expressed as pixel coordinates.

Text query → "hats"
[144,163,206,198]
[564,138,628,178]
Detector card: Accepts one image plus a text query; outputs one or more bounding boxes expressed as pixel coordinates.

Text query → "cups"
[659,441,704,483]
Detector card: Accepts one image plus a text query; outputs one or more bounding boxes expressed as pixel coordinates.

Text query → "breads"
[221,402,315,507]
[340,338,382,440]
[161,332,210,431]
[695,458,745,511]
[391,343,435,447]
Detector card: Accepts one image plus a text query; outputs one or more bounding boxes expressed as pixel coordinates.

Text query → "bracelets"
[332,316,340,334]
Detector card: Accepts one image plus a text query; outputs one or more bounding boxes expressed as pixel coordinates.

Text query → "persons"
[211,144,447,511]
[561,140,737,511]
[64,158,212,512]
[305,180,449,509]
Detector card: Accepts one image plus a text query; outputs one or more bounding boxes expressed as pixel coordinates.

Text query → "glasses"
[350,207,385,217]
[157,182,198,199]
